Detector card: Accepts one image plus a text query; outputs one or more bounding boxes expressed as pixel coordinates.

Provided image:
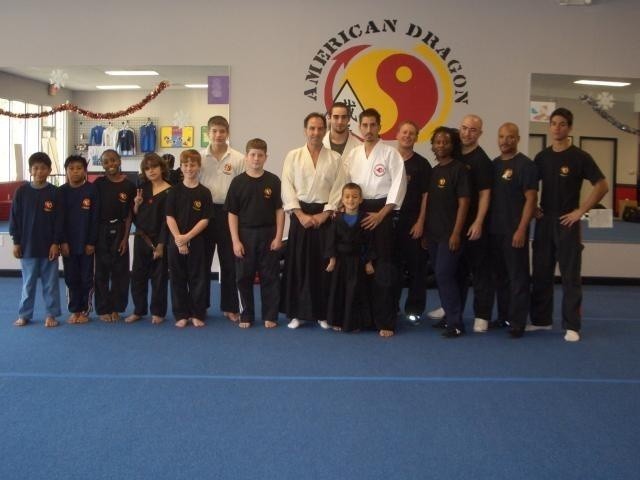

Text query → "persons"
[93,149,137,322]
[59,155,100,324]
[164,148,216,328]
[8,151,65,328]
[124,155,174,324]
[162,153,180,185]
[222,137,286,329]
[280,100,609,342]
[196,115,246,322]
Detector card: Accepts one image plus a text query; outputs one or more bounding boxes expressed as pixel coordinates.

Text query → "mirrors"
[530,73,639,244]
[0,66,231,236]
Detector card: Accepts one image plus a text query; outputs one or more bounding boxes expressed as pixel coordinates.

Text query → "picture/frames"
[161,126,194,148]
[580,136,617,216]
[201,126,209,147]
[567,136,573,144]
[529,134,545,207]
